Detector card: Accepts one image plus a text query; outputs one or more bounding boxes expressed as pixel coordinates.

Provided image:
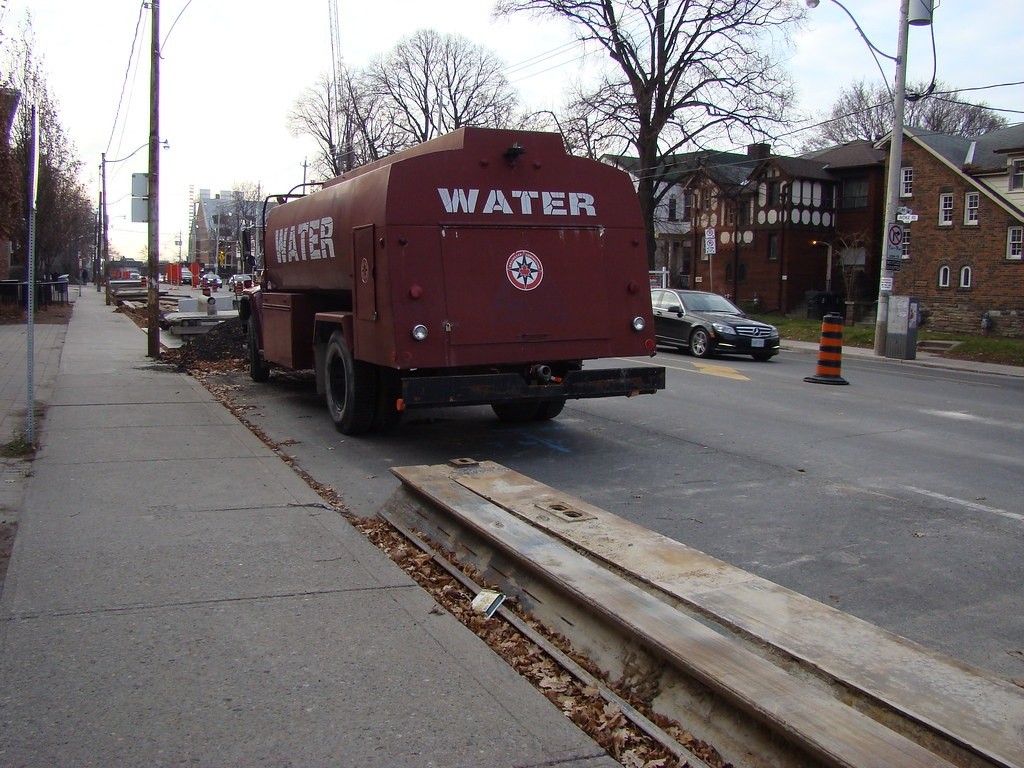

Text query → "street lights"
[808,0,907,358]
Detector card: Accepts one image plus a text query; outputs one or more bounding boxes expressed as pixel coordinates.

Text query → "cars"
[228,274,252,292]
[181,268,193,284]
[650,288,780,361]
[201,271,223,288]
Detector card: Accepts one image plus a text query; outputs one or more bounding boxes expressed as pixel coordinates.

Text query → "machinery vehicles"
[238,127,666,437]
